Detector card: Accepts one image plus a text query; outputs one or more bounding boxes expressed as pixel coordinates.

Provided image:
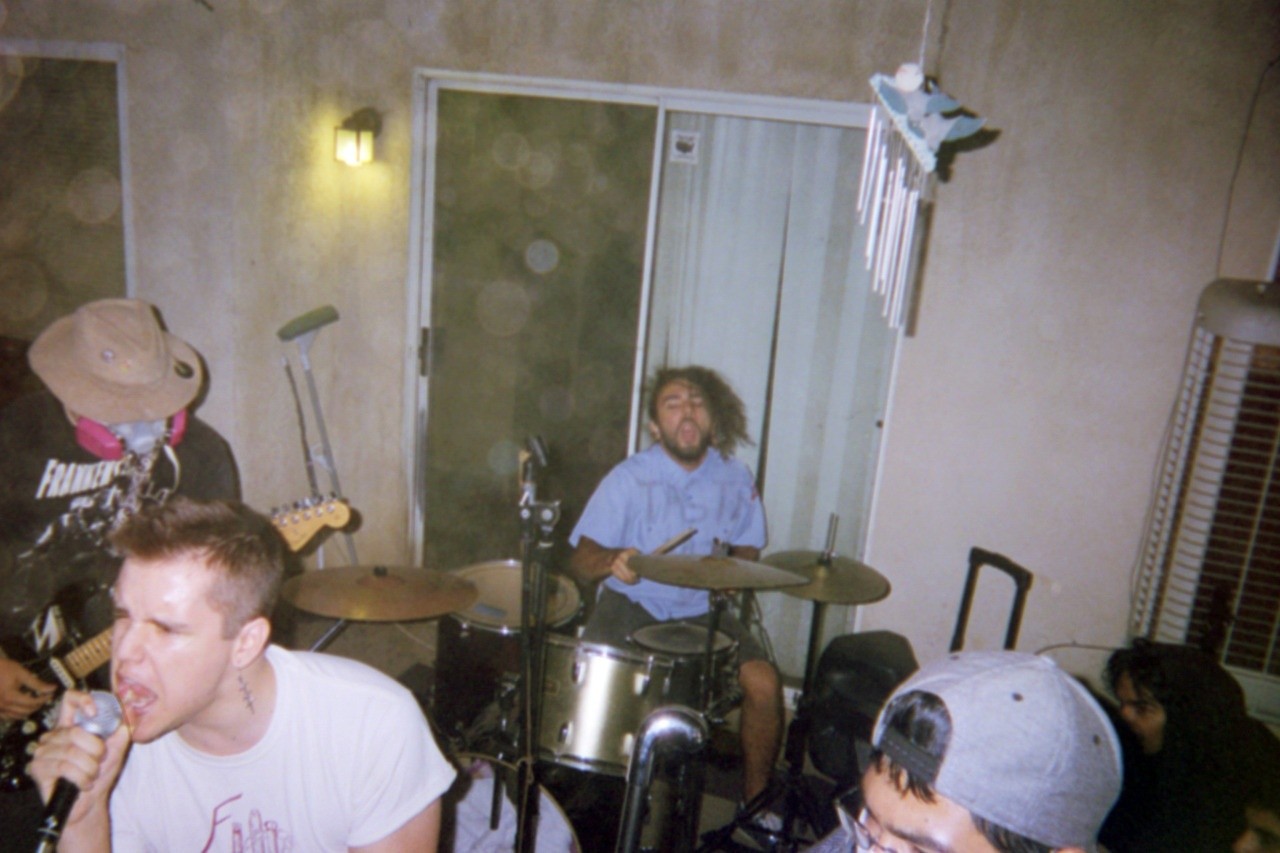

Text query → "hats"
[874,649,1124,850]
[26,297,203,423]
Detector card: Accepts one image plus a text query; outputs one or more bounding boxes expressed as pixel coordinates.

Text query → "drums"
[534,634,672,779]
[445,558,583,685]
[630,619,742,715]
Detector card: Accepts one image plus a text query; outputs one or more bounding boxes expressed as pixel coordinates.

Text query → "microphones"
[525,431,551,468]
[35,689,123,853]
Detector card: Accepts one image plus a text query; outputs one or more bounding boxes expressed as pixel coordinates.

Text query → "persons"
[804,639,1280,853]
[564,363,781,832]
[21,500,460,853]
[0,297,239,853]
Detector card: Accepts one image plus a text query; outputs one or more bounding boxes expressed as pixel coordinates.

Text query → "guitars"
[0,493,349,767]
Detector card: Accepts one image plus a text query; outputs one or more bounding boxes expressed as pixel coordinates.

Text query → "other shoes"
[734,803,793,853]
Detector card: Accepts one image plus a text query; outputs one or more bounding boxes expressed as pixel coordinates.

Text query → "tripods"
[692,598,832,851]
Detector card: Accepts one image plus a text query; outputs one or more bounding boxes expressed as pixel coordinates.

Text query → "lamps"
[332,106,384,169]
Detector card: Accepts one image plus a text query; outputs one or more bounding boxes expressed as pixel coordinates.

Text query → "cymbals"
[282,563,475,620]
[631,553,811,595]
[762,549,892,606]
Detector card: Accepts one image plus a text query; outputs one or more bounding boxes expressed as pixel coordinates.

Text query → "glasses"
[834,786,895,853]
